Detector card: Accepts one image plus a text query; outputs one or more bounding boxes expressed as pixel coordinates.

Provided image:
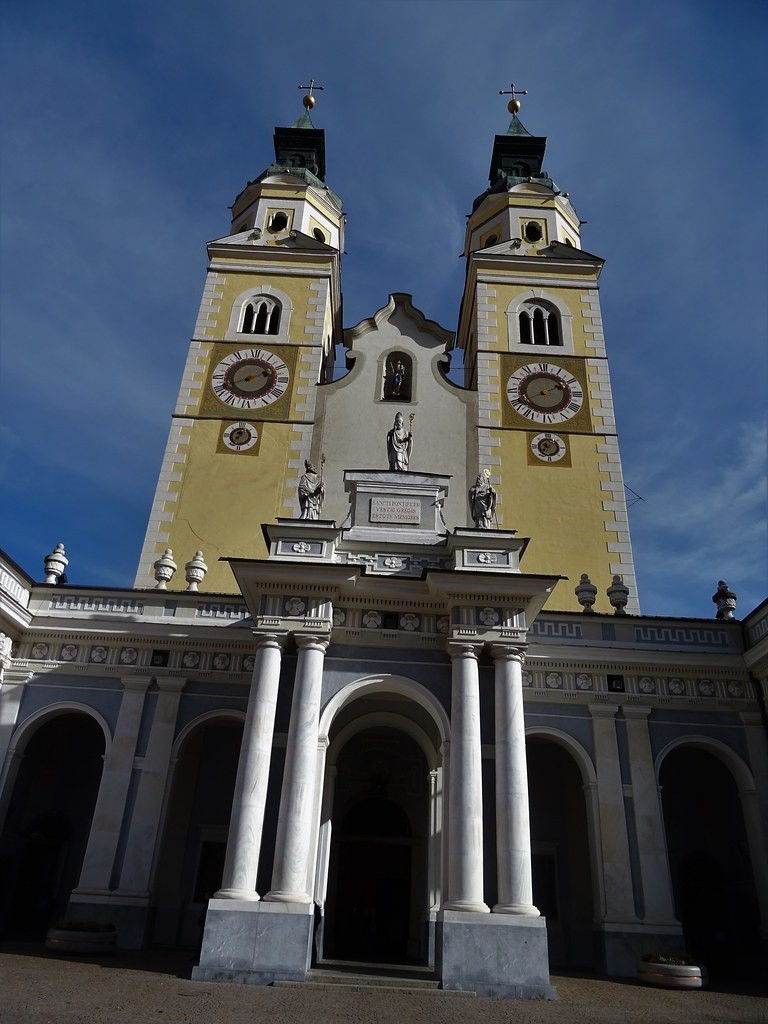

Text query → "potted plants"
[37,916,117,953]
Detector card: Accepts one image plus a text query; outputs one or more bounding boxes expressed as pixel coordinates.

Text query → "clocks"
[216,419,265,456]
[501,352,592,432]
[527,431,572,469]
[198,342,299,420]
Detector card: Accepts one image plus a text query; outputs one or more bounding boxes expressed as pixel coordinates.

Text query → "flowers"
[643,950,694,965]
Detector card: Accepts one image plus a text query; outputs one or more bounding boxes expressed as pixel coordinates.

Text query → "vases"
[636,960,710,990]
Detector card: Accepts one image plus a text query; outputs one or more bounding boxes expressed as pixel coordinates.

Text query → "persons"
[298,460,326,520]
[469,473,497,529]
[387,412,413,471]
